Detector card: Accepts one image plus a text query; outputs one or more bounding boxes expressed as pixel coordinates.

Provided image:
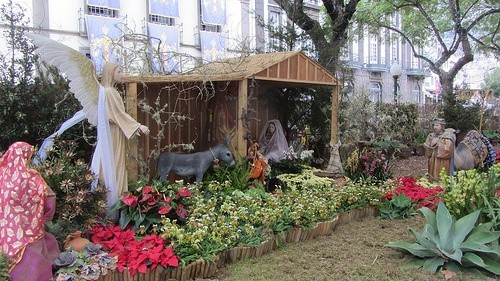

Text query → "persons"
[256,119,287,157]
[424,119,457,185]
[1,142,60,281]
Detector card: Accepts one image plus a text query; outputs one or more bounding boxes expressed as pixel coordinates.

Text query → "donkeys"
[157,137,235,185]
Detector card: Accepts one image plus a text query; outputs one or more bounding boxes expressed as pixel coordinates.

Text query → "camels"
[443,128,488,171]
